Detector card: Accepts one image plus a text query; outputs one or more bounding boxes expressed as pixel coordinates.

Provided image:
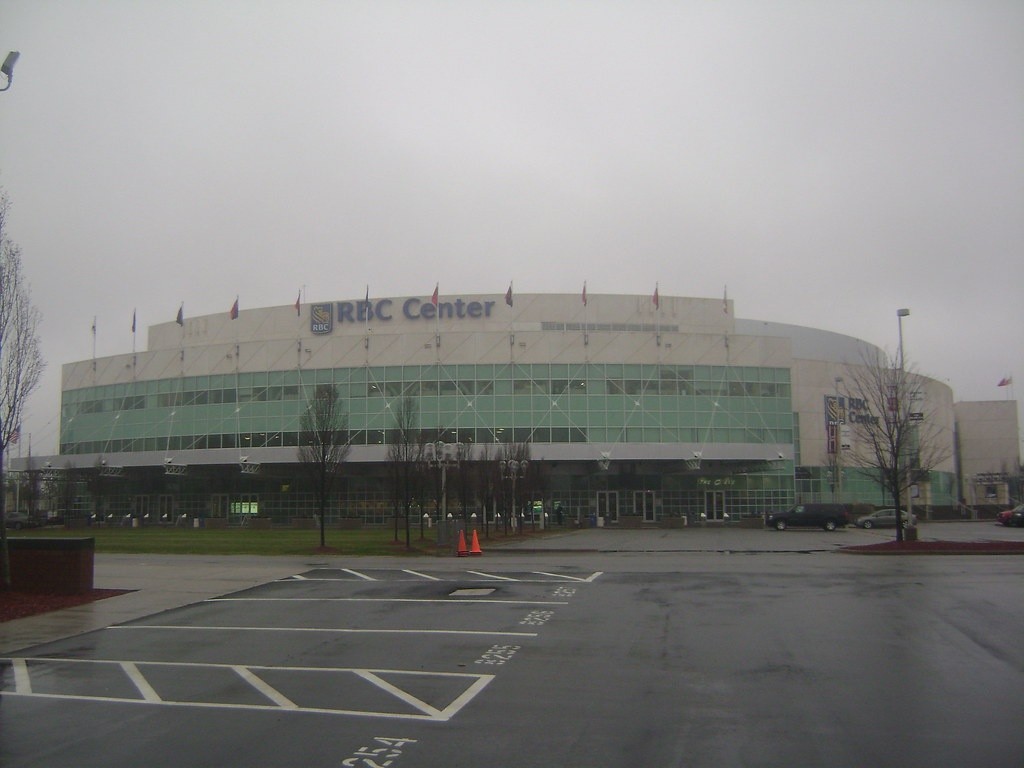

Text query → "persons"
[557,503,563,526]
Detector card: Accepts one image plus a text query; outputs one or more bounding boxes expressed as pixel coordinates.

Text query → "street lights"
[896,308,918,541]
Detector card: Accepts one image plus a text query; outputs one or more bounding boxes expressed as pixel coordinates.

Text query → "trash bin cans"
[193,516,199,528]
[604,512,612,525]
[132,516,138,528]
[590,515,596,526]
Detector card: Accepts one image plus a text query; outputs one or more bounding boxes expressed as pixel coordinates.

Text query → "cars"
[853,509,917,530]
[995,505,1024,528]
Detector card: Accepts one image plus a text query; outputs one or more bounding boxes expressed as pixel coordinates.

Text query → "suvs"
[766,503,849,531]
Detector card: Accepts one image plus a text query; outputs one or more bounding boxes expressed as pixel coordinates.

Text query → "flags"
[582,285,587,306]
[176,305,183,327]
[431,286,438,307]
[998,377,1013,386]
[365,289,368,307]
[652,287,659,309]
[295,294,301,316]
[230,299,238,320]
[132,312,135,332]
[723,289,727,313]
[505,286,513,307]
[9,424,20,444]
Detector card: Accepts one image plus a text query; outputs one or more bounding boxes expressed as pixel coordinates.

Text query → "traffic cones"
[456,530,470,557]
[468,530,482,557]
